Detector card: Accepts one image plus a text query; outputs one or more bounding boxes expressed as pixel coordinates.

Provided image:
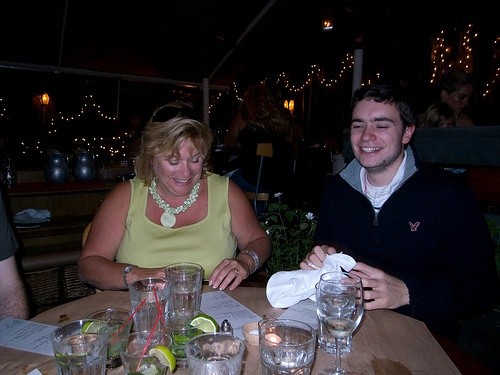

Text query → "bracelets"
[238,249,259,274]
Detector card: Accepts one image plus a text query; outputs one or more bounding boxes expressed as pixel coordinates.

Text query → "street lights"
[39,91,50,144]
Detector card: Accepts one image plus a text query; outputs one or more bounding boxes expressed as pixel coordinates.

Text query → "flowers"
[258,191,318,275]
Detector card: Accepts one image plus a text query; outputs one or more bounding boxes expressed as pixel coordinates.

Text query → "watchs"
[122,265,138,288]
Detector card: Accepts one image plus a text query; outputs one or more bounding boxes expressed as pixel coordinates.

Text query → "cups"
[50,319,110,375]
[187,333,245,375]
[87,308,133,369]
[129,278,169,344]
[315,280,356,354]
[260,319,316,375]
[165,262,203,330]
[171,310,206,361]
[119,331,172,375]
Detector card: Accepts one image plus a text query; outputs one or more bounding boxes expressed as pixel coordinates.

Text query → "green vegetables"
[167,333,194,358]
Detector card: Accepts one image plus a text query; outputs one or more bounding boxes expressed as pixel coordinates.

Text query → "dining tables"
[0,285,464,375]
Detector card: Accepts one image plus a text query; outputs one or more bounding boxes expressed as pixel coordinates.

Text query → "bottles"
[219,320,233,336]
[4,159,16,189]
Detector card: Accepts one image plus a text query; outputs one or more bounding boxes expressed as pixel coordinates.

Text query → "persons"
[0,192,29,323]
[298,85,500,343]
[417,67,476,128]
[78,101,272,291]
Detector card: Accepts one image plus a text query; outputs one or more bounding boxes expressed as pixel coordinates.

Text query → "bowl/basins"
[243,322,274,346]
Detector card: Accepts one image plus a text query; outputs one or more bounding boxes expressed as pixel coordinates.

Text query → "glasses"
[152,106,202,123]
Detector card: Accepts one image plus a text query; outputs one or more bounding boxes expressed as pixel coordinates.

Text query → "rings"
[232,269,239,273]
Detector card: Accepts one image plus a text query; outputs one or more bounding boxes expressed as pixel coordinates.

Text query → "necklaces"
[148,177,201,227]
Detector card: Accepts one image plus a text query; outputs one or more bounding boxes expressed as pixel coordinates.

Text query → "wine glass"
[319,271,364,375]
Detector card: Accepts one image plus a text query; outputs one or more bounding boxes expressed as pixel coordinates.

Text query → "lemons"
[82,321,99,333]
[189,314,219,334]
[149,345,176,372]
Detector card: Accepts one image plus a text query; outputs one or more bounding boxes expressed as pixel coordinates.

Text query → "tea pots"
[67,147,95,183]
[43,149,67,183]
